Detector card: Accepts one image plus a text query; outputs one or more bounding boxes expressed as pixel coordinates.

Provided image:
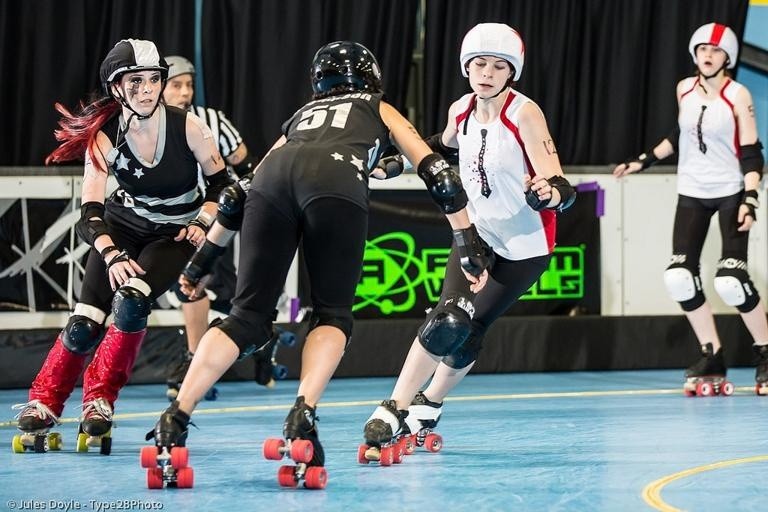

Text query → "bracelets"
[99,245,120,261]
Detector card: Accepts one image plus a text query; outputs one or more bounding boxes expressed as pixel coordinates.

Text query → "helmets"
[162,56,196,81]
[460,23,524,82]
[310,40,382,95]
[100,39,168,86]
[689,23,738,69]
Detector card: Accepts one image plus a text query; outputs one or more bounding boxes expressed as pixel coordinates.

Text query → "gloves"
[524,177,553,212]
[739,191,760,221]
[625,147,659,174]
[185,218,210,233]
[182,238,227,286]
[453,225,495,277]
[106,249,129,275]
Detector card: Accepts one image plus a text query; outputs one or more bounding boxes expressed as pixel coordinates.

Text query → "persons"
[13,38,229,455]
[358,22,577,466]
[163,55,297,402]
[139,40,495,490]
[613,22,767,398]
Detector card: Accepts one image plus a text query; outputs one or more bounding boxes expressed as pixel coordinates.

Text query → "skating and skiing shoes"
[12,400,61,453]
[74,397,113,455]
[403,392,444,455]
[141,402,194,491]
[685,344,734,397]
[753,343,768,396]
[358,401,410,467]
[264,396,327,490]
